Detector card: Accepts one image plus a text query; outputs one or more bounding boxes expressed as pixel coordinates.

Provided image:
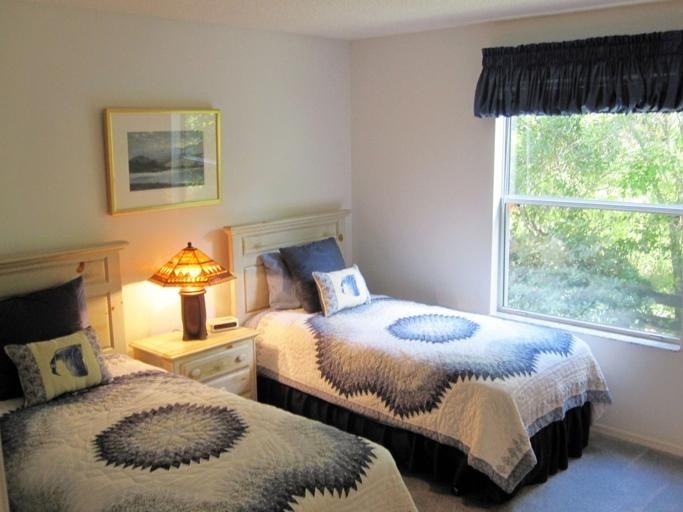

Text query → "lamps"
[142,239,239,343]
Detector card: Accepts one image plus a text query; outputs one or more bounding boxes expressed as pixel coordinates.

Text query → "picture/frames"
[100,105,222,215]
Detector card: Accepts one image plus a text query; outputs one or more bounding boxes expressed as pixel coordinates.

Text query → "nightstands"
[128,326,261,406]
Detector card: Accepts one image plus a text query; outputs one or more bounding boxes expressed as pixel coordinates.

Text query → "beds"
[220,208,615,510]
[1,237,434,511]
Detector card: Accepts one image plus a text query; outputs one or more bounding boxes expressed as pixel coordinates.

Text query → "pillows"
[0,325,116,410]
[257,249,305,313]
[276,235,353,315]
[308,260,372,317]
[0,272,90,399]
